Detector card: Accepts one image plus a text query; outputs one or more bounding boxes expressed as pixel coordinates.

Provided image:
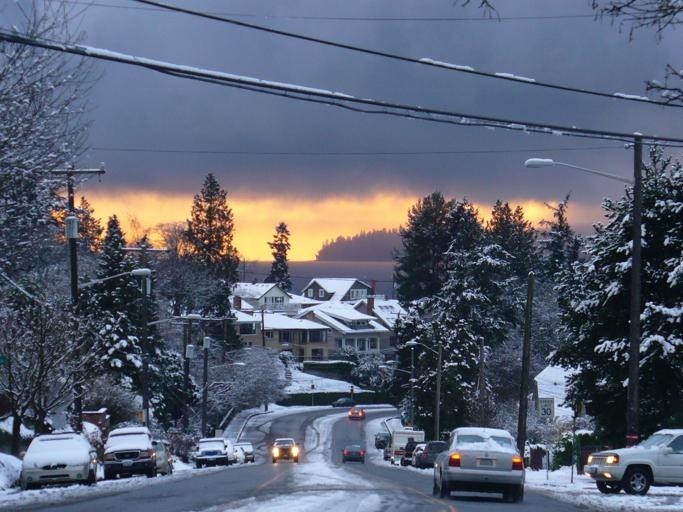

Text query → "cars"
[583,427,683,496]
[375,426,525,503]
[19,426,173,490]
[193,438,255,468]
[332,398,356,408]
[348,407,366,420]
[342,445,366,464]
[271,438,299,463]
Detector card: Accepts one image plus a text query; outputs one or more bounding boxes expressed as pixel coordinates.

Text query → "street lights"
[522,130,645,448]
[377,341,444,441]
[65,215,152,433]
[142,278,202,432]
